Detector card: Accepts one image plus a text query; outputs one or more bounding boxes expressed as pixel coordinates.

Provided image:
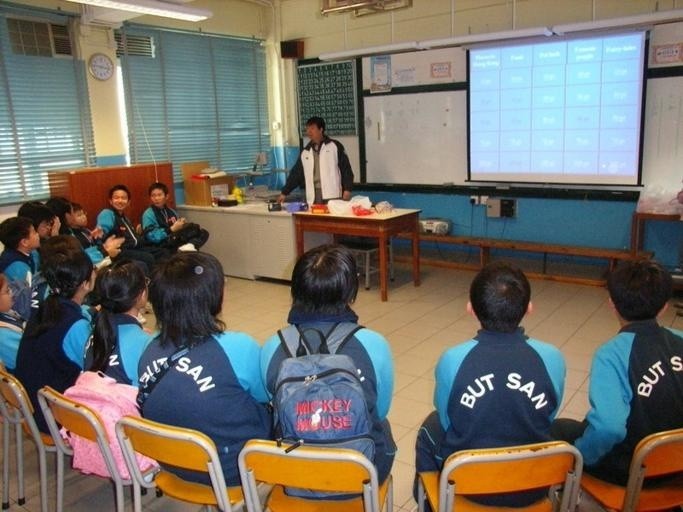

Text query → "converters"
[470,199,475,204]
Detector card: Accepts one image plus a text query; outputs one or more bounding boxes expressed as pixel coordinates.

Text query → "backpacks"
[60,370,162,480]
[9,279,32,323]
[271,352,378,499]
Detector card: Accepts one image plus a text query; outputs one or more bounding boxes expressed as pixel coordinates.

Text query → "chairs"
[338,202,395,290]
[2,372,59,510]
[416,442,580,511]
[236,439,395,510]
[114,416,249,510]
[39,385,155,512]
[577,426,682,511]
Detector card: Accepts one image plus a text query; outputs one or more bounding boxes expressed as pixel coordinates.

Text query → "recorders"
[418,219,451,235]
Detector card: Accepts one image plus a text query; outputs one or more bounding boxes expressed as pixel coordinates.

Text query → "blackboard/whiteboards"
[295,57,359,138]
[299,17,683,202]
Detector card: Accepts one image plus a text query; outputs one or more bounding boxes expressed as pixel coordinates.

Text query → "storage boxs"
[181,162,235,207]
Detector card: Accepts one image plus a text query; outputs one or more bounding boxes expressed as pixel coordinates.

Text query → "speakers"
[280,41,304,59]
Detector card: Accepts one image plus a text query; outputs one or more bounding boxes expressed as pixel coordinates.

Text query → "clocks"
[88,52,113,81]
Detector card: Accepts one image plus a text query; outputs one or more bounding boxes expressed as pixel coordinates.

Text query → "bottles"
[247,181,254,202]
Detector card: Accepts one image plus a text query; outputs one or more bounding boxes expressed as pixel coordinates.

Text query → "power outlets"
[272,122,280,130]
[470,196,479,206]
[481,195,489,204]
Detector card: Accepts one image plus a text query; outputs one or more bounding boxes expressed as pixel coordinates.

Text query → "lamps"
[250,153,268,189]
[66,0,214,22]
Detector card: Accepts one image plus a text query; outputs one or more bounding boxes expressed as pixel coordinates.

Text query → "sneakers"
[178,244,198,254]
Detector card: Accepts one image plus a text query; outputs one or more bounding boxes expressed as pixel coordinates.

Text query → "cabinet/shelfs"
[47,160,174,232]
[177,184,334,281]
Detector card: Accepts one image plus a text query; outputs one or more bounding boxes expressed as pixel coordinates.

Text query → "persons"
[552,252,682,489]
[275,116,354,204]
[258,243,398,501]
[0,185,272,488]
[413,261,567,512]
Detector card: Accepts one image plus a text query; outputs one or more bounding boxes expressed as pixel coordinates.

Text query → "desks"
[634,192,683,289]
[293,207,422,300]
